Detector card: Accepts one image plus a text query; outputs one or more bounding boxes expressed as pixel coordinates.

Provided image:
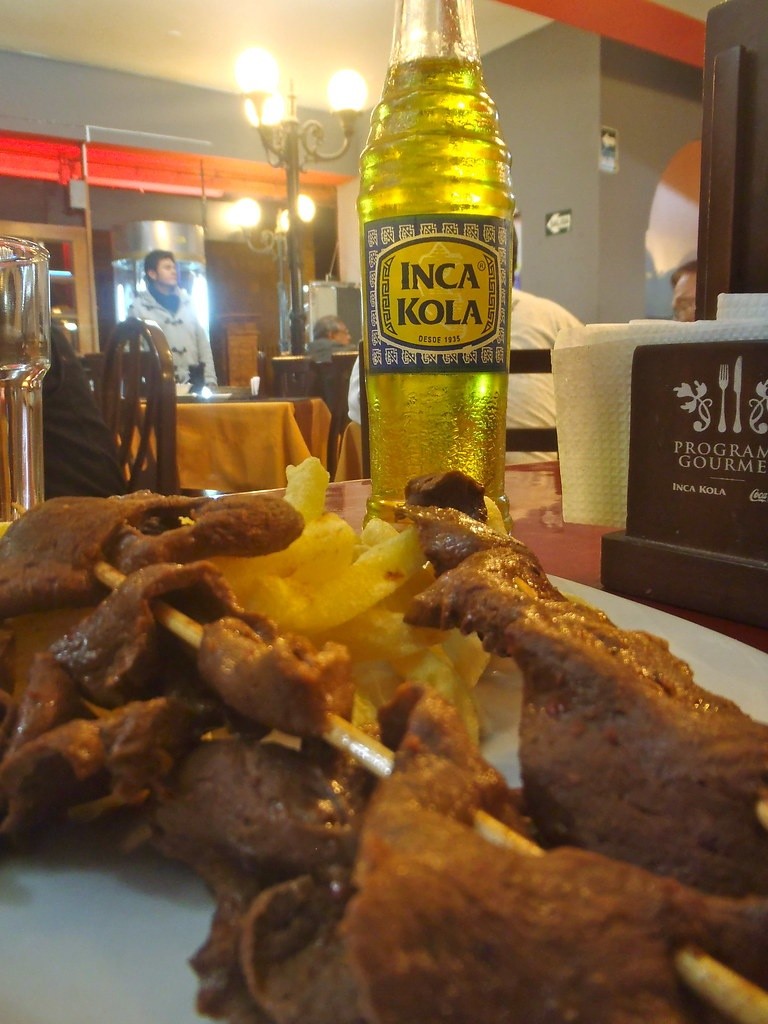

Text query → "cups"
[0,233,52,535]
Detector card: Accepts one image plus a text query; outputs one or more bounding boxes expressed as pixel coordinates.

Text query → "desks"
[334,421,363,482]
[116,397,331,497]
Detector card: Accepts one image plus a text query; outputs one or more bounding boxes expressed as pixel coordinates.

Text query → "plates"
[202,392,232,403]
[2,572,767,1024]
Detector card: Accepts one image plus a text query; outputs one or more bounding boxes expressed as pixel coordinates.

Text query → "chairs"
[256,351,268,399]
[98,317,180,497]
[358,341,560,479]
[271,350,358,471]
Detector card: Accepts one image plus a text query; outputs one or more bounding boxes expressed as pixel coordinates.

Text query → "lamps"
[226,45,371,356]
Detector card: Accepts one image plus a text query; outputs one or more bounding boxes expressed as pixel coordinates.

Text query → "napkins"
[550,292,768,529]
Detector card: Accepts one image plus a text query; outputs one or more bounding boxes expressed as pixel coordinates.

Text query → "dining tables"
[0,461,768,651]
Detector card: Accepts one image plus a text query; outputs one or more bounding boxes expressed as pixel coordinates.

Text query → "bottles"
[360,0,519,527]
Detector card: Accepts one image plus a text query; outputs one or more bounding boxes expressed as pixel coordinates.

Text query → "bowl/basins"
[176,382,193,396]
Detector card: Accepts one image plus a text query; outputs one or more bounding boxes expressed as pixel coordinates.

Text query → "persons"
[347,224,583,497]
[130,248,220,397]
[300,315,352,424]
[669,260,699,321]
[41,320,126,496]
